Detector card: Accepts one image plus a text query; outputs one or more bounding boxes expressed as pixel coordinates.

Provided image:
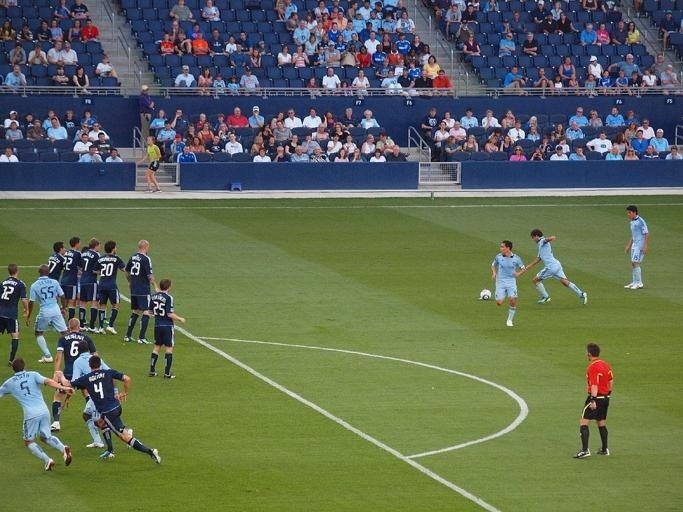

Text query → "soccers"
[480,289,491,301]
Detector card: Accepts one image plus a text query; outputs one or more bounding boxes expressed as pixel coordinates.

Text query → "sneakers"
[507,320,513,327]
[8,359,15,367]
[86,442,104,448]
[80,325,117,335]
[38,356,54,363]
[150,448,161,464]
[573,448,591,458]
[538,296,552,304]
[124,336,153,344]
[624,283,643,289]
[51,423,61,431]
[145,189,162,192]
[595,448,609,455]
[149,372,176,378]
[62,446,72,466]
[580,292,587,304]
[126,428,133,449]
[44,458,55,471]
[99,451,114,458]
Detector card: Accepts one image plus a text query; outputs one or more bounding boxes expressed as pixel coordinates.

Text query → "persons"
[1,109,123,163]
[272,1,455,95]
[624,206,649,289]
[25,265,68,363]
[573,344,613,459]
[490,240,526,328]
[48,236,126,336]
[0,264,28,366]
[136,84,162,191]
[524,229,587,305]
[159,0,271,96]
[444,0,682,96]
[149,108,408,163]
[1,1,121,95]
[419,105,682,160]
[1,318,161,471]
[124,240,185,378]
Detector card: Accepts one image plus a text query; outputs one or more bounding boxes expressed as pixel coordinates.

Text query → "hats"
[403,67,408,70]
[539,0,544,5]
[175,134,181,138]
[657,129,663,133]
[590,56,597,61]
[556,145,562,150]
[141,85,149,92]
[253,106,259,111]
[183,65,188,70]
[328,41,335,46]
[398,32,405,36]
[504,19,508,23]
[35,42,41,47]
[666,10,672,13]
[10,111,17,114]
[356,10,362,14]
